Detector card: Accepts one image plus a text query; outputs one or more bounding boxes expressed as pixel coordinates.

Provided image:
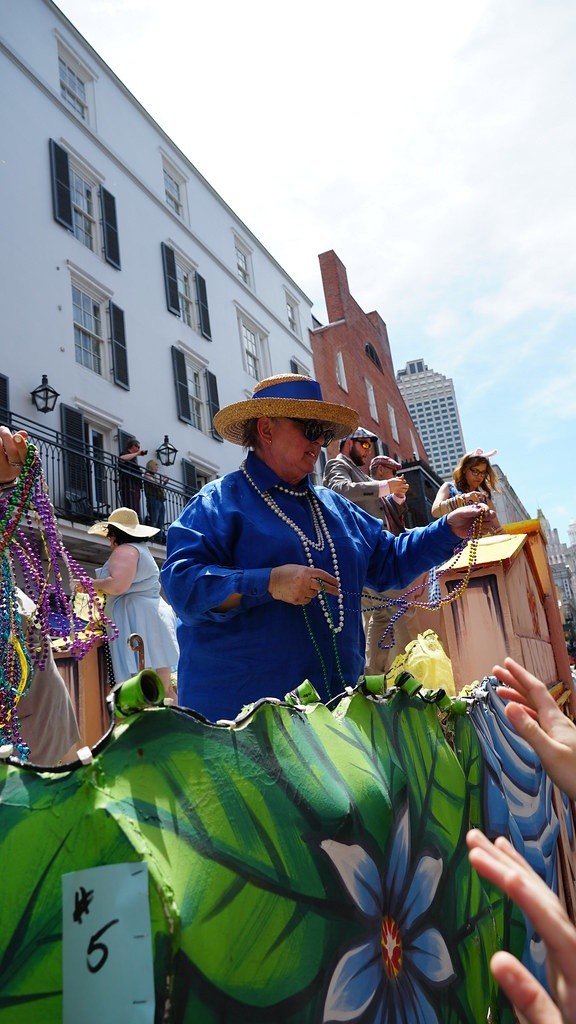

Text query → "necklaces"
[238,459,485,708]
[0,441,118,762]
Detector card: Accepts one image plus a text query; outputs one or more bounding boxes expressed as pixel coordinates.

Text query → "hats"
[370,455,401,470]
[212,373,360,441]
[87,507,159,537]
[339,426,378,446]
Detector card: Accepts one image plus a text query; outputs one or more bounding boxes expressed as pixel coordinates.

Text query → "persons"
[158,373,496,723]
[432,449,506,535]
[323,427,409,676]
[466,829,576,1024]
[0,424,81,766]
[72,507,180,704]
[493,657,576,801]
[144,460,169,542]
[118,440,144,515]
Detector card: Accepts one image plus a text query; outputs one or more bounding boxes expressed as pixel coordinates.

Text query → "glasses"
[384,465,396,474]
[357,440,372,450]
[286,416,333,447]
[470,468,488,479]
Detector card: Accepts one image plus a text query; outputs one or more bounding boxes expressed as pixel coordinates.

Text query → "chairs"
[146,516,171,542]
[65,490,111,517]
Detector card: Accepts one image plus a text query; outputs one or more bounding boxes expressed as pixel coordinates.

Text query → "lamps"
[32,375,60,413]
[156,435,178,466]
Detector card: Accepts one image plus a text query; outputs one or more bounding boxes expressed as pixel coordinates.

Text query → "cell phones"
[140,450,147,456]
